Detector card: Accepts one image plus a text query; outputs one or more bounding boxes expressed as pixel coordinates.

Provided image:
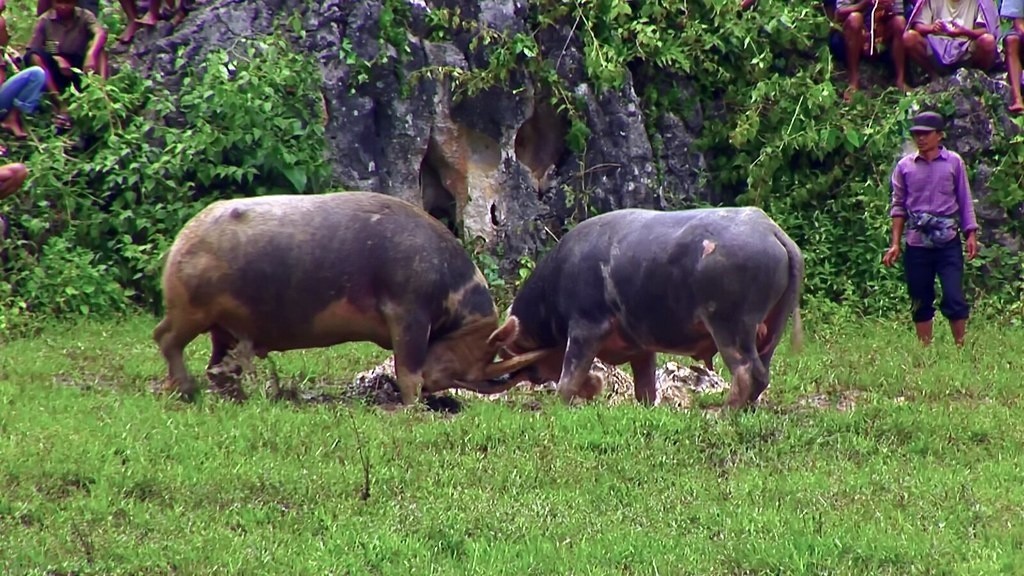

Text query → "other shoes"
[54,109,72,129]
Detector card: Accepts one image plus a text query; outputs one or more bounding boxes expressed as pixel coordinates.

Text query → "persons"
[0,0,205,273]
[883,112,979,348]
[736,0,1024,110]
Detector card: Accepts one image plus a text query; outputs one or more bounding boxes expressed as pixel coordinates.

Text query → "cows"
[491,203,808,410]
[148,187,554,421]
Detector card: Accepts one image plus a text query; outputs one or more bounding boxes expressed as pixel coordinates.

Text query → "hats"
[908,111,945,131]
